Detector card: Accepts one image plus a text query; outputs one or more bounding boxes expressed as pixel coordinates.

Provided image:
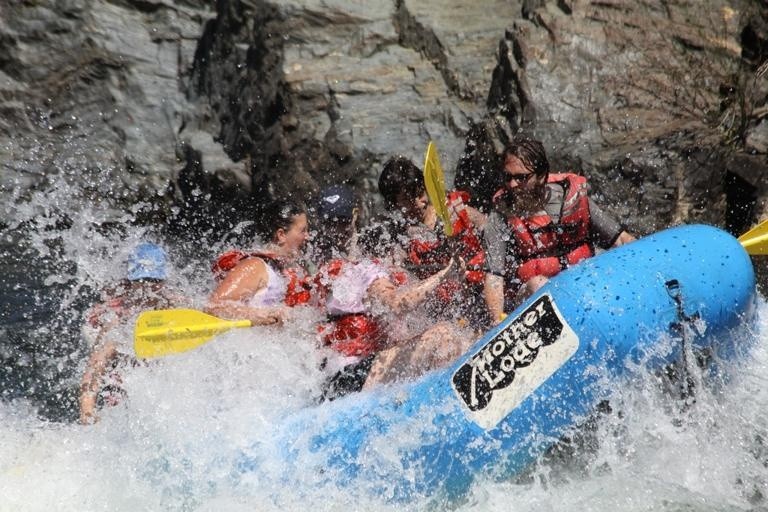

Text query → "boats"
[127,223,759,503]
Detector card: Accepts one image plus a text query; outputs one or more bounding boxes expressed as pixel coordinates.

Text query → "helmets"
[126,242,173,281]
[316,186,359,224]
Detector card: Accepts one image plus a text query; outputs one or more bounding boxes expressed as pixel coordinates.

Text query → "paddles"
[737,221,768,255]
[134,308,280,358]
[423,140,471,298]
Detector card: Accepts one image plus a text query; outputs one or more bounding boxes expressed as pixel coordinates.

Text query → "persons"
[319,153,486,399]
[482,139,638,325]
[209,197,316,325]
[79,243,183,425]
